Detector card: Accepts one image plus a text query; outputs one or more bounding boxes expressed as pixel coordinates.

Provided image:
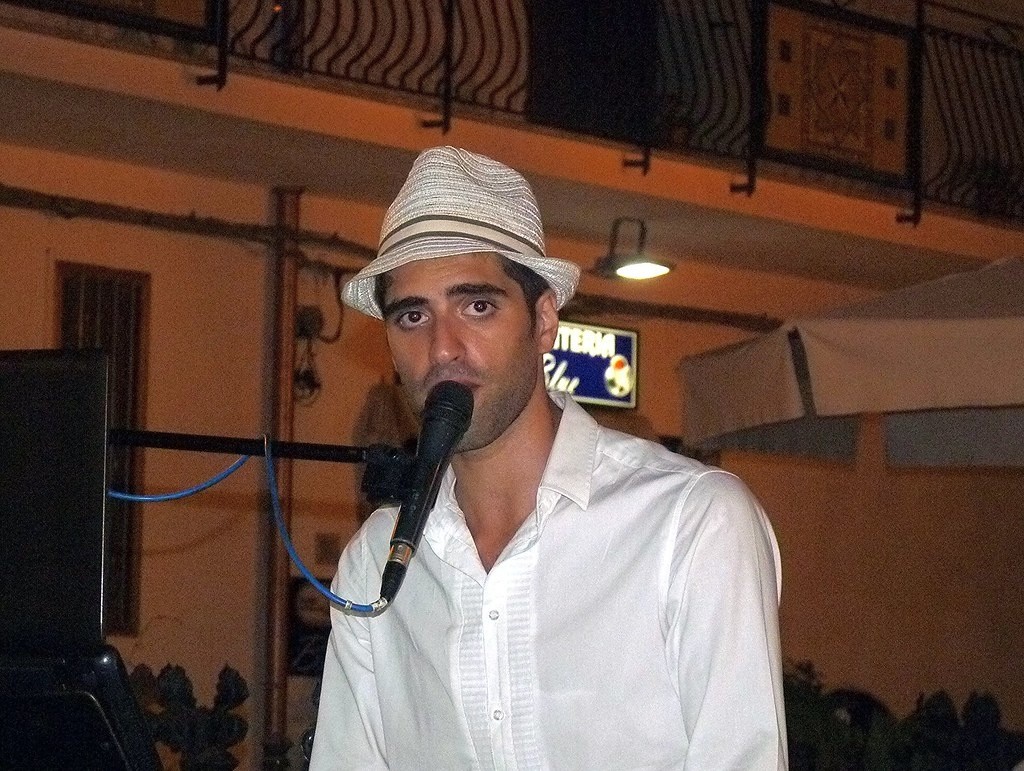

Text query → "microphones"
[381,380,474,602]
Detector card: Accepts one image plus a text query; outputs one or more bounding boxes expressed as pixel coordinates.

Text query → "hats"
[341,146,582,324]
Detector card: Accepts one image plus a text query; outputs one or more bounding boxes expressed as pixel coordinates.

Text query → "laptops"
[0,346,164,771]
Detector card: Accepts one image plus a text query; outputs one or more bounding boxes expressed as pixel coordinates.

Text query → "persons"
[308,145,790,771]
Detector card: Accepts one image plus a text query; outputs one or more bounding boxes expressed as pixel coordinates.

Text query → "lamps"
[594,216,673,280]
